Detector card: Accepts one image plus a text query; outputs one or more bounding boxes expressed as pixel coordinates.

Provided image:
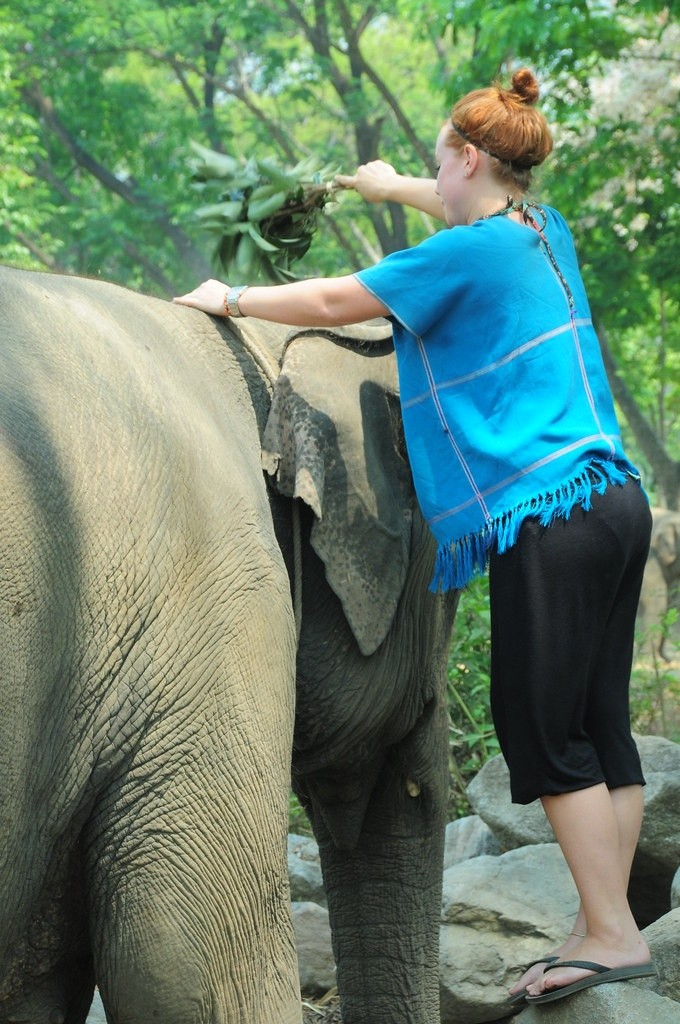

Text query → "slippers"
[503,954,561,1007]
[524,954,659,1005]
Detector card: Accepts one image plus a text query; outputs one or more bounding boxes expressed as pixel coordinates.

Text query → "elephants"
[2,267,468,1023]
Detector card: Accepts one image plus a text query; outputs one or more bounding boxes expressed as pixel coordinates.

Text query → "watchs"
[226,285,248,318]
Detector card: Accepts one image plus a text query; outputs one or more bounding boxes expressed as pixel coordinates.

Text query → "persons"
[172,65,658,1005]
